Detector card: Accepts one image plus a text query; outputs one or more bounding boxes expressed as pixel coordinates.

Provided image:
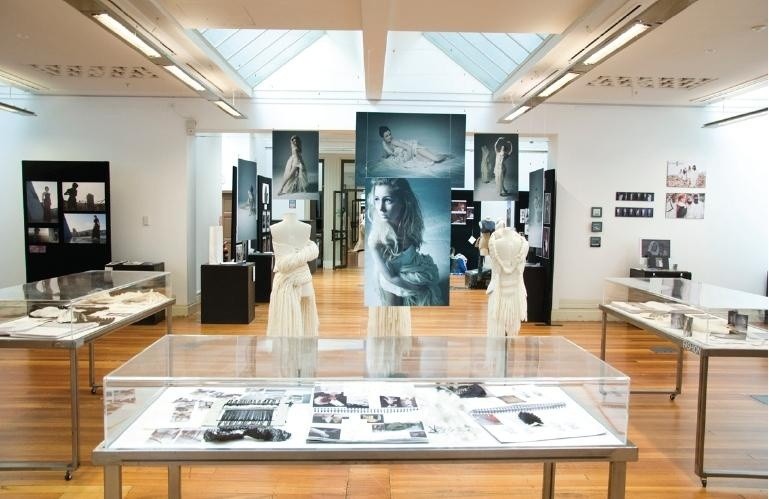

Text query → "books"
[305,383,430,445]
[451,385,607,444]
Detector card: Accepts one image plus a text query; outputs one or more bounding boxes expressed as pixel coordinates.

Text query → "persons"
[277,135,311,196]
[40,185,52,222]
[492,136,513,196]
[375,124,448,165]
[366,177,446,306]
[484,224,530,337]
[680,164,698,188]
[31,228,42,243]
[63,183,79,211]
[479,143,491,184]
[90,214,102,240]
[246,184,256,216]
[675,194,705,219]
[268,210,320,338]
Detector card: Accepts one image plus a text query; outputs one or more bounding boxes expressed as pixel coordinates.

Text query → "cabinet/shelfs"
[91,334,640,499]
[629,268,692,331]
[596,277,768,487]
[0,269,175,480]
[200,262,257,325]
[247,253,275,302]
[524,266,546,322]
[112,261,164,325]
